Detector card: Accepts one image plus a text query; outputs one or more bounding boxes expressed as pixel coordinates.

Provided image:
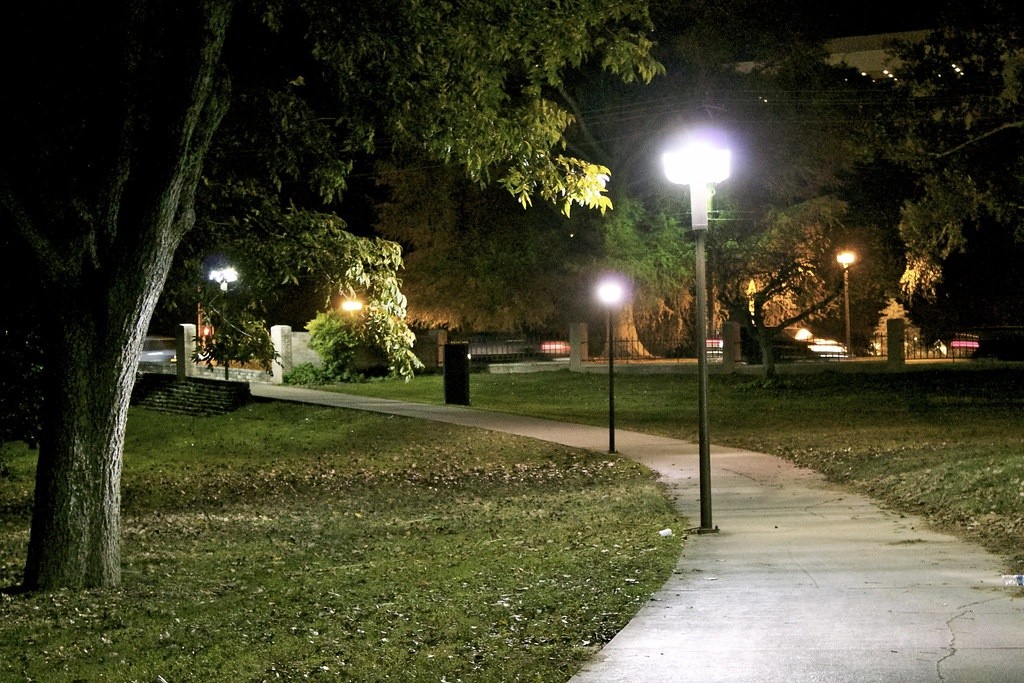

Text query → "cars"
[139,335,177,365]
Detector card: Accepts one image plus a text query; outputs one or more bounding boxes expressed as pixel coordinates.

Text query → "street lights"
[208,269,239,381]
[657,139,734,538]
[836,250,855,357]
[603,282,621,453]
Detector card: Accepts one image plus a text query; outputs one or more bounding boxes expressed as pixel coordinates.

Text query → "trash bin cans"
[443,341,470,406]
